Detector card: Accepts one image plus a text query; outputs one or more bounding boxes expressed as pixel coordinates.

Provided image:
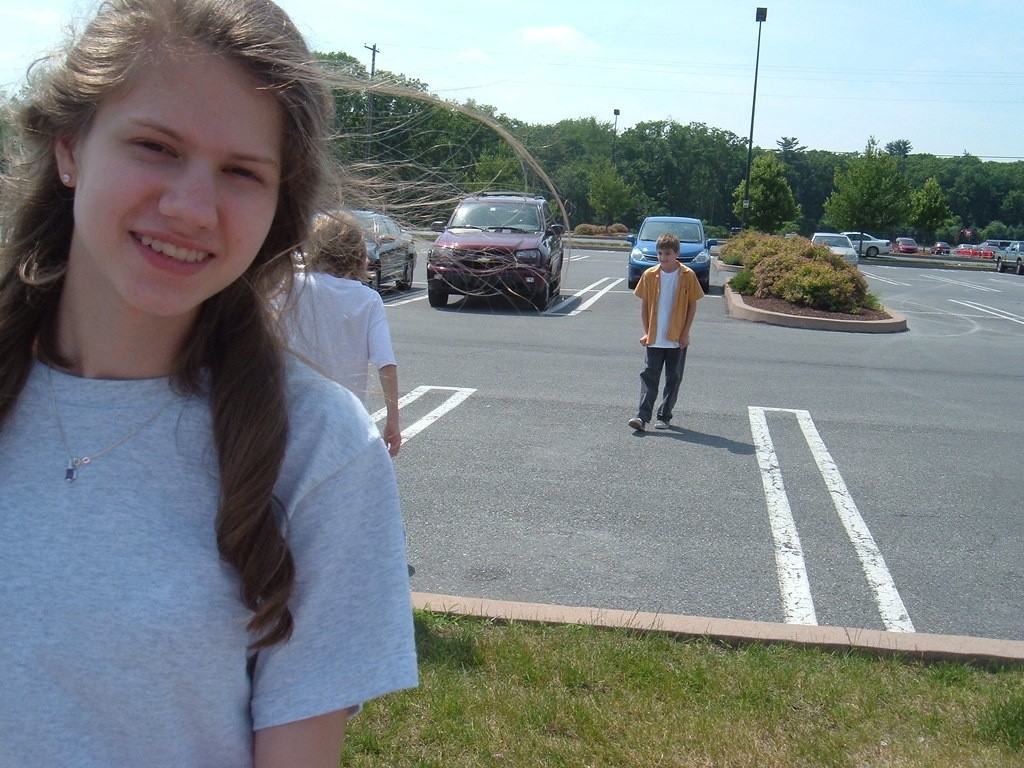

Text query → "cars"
[930,242,950,255]
[840,232,891,257]
[995,241,1024,275]
[289,210,417,294]
[892,237,919,253]
[811,232,858,266]
[626,217,718,292]
[953,240,1015,260]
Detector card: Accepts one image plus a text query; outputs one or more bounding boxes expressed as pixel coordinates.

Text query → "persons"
[264,210,403,457]
[628,232,705,432]
[0,0,418,768]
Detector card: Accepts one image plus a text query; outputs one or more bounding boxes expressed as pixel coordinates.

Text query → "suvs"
[427,192,566,311]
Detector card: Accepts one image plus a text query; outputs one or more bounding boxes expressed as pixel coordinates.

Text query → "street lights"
[605,109,621,232]
[741,8,769,231]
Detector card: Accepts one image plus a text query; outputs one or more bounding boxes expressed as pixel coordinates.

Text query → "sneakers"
[628,417,646,432]
[653,420,671,429]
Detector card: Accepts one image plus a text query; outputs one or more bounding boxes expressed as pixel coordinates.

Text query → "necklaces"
[46,356,176,482]
